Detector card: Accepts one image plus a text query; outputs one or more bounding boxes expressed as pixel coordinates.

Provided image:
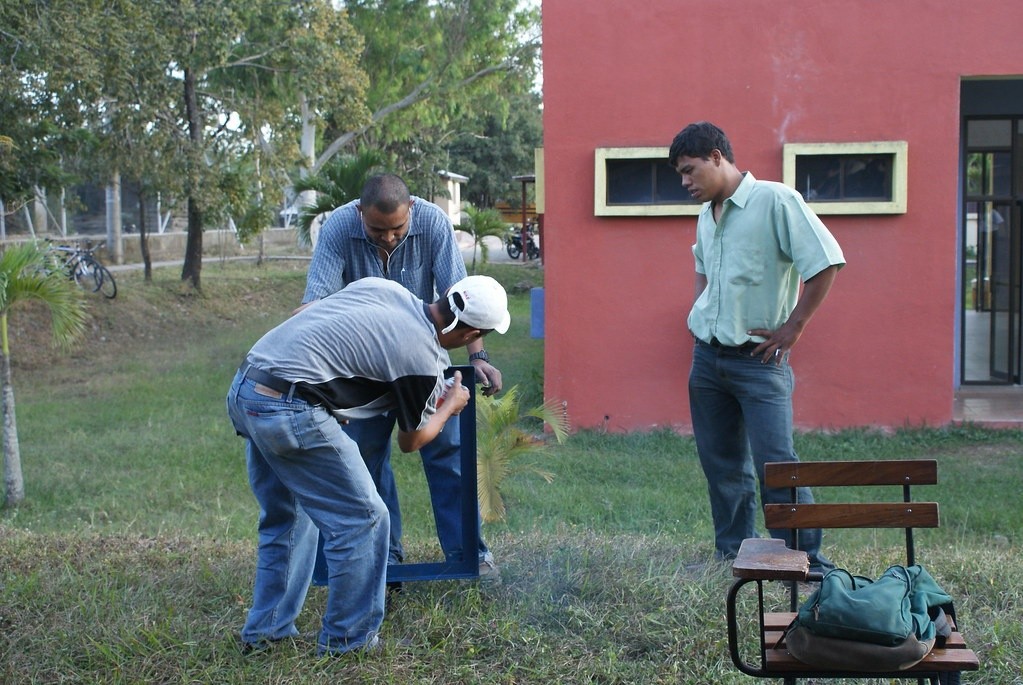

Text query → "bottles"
[435,377,455,433]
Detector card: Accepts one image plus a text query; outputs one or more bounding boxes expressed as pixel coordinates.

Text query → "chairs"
[724,460,980,685]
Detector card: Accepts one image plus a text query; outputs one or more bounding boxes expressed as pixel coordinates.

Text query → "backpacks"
[773,565,957,673]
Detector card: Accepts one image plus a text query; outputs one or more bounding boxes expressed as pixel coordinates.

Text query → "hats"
[441,275,511,335]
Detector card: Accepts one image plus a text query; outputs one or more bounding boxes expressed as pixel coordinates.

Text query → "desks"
[725,538,808,668]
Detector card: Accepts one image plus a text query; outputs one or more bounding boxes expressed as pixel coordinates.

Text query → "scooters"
[506,219,535,260]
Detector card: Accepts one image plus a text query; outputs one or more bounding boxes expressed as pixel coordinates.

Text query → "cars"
[278,206,299,227]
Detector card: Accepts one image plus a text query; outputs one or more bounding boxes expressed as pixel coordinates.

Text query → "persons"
[302,175,502,603]
[669,120,846,591]
[226,276,510,659]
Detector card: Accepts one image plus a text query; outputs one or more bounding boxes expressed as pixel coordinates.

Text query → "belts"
[240,358,313,402]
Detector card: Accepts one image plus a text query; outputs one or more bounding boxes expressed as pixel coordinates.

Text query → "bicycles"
[30,236,117,299]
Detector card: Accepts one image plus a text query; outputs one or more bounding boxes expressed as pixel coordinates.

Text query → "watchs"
[469,349,490,363]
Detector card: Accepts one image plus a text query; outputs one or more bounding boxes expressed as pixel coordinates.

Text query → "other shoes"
[477,551,500,584]
[370,637,411,656]
[243,631,317,655]
